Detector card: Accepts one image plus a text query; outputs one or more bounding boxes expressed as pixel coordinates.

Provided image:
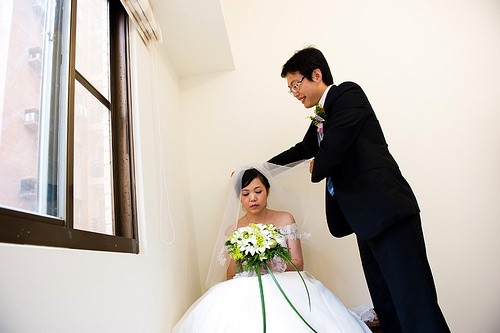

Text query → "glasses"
[288,75,306,95]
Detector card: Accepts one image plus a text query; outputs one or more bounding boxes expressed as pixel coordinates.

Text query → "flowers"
[309,104,325,126]
[225,222,317,333]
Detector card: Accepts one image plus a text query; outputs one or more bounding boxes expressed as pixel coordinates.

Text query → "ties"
[317,129,334,196]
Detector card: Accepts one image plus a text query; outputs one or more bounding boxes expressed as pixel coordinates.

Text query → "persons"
[266,45,450,333]
[170,167,372,333]
